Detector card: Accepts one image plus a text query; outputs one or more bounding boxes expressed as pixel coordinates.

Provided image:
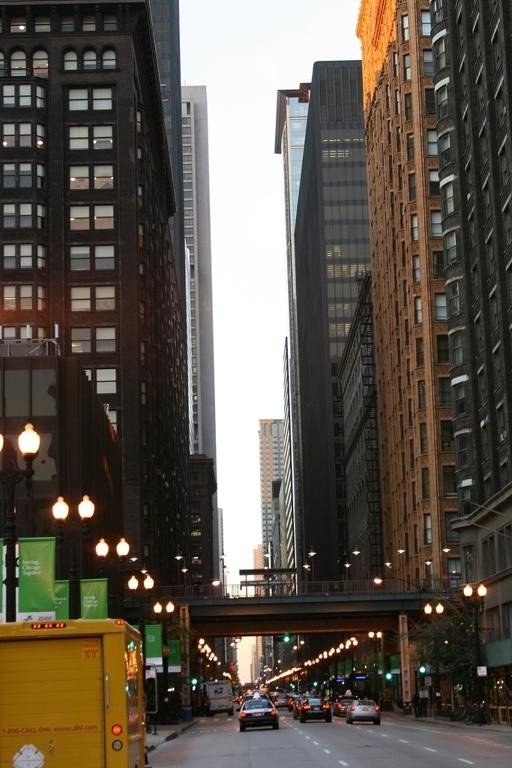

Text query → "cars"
[238,690,381,731]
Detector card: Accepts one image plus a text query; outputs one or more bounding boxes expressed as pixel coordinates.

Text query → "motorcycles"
[464,700,491,725]
[0,618,149,768]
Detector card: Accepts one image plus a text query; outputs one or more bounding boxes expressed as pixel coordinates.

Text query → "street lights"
[304,631,382,705]
[190,638,221,678]
[424,584,487,723]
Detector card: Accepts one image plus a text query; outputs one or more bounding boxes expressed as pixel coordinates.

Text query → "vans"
[200,679,234,715]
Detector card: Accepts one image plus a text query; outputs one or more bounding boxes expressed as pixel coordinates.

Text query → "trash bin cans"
[411,693,428,718]
[183,705,193,722]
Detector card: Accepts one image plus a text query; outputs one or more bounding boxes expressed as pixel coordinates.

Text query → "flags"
[0,534,183,673]
